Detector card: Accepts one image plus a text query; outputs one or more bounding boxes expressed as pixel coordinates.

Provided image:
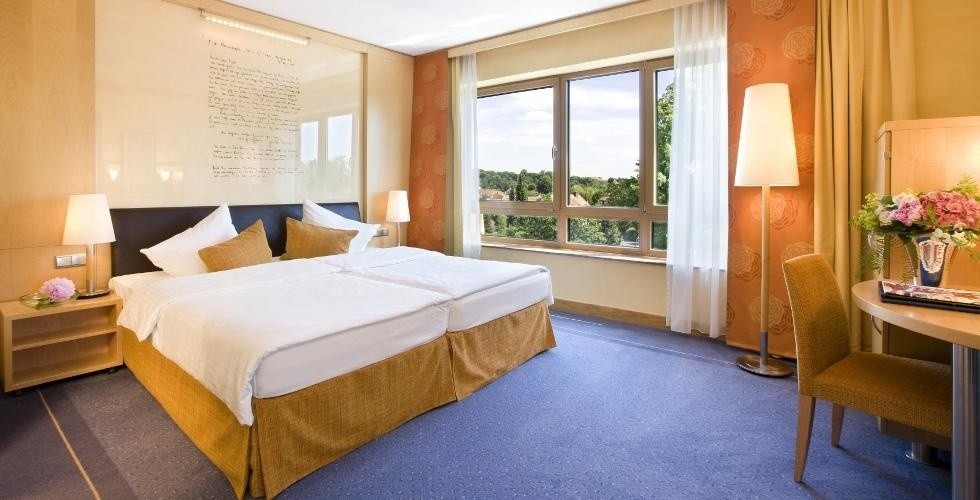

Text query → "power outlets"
[56,254,87,268]
[373,229,388,236]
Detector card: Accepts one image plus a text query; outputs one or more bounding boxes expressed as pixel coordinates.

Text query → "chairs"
[782,254,953,486]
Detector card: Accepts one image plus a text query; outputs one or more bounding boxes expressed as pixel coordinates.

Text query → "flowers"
[851,178,980,281]
[38,277,73,304]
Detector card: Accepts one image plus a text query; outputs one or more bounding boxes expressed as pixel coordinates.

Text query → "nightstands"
[0,290,123,397]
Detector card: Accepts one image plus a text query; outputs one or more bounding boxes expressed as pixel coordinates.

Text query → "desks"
[851,280,980,499]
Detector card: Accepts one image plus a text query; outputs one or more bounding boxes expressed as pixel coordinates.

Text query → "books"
[879,281,975,312]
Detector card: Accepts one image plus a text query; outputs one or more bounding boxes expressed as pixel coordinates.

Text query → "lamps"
[386,190,411,248]
[734,81,799,377]
[62,193,116,299]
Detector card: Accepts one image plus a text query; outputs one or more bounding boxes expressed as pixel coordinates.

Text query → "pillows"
[300,198,380,253]
[279,216,358,260]
[199,218,272,271]
[141,202,238,275]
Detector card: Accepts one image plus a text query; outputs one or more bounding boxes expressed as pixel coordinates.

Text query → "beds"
[109,202,558,500]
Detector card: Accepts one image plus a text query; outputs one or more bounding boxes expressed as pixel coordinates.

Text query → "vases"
[19,291,78,309]
[919,237,954,289]
